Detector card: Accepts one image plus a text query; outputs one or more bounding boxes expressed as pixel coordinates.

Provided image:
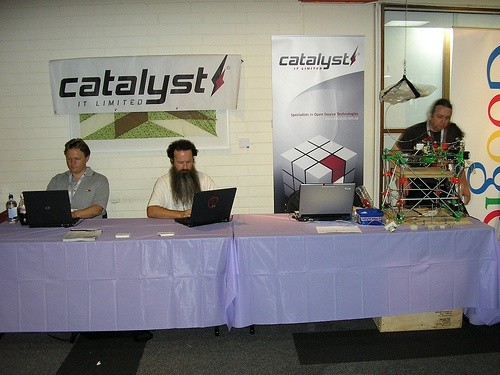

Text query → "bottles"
[17,193,23,220]
[355,186,374,210]
[20,198,28,225]
[6,193,18,223]
[412,144,429,167]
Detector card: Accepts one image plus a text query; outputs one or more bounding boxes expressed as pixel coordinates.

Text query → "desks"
[0,213,500,336]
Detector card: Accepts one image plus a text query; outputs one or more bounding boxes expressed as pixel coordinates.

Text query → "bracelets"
[74,211,80,218]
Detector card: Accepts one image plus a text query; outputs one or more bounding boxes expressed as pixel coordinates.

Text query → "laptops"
[23,190,80,228]
[295,183,358,220]
[175,188,236,227]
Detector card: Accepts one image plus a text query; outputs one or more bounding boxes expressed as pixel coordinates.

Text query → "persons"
[147,139,218,219]
[389,99,471,216]
[46,138,109,221]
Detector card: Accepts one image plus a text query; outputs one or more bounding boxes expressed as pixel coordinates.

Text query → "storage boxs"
[372,309,463,333]
[356,208,386,225]
